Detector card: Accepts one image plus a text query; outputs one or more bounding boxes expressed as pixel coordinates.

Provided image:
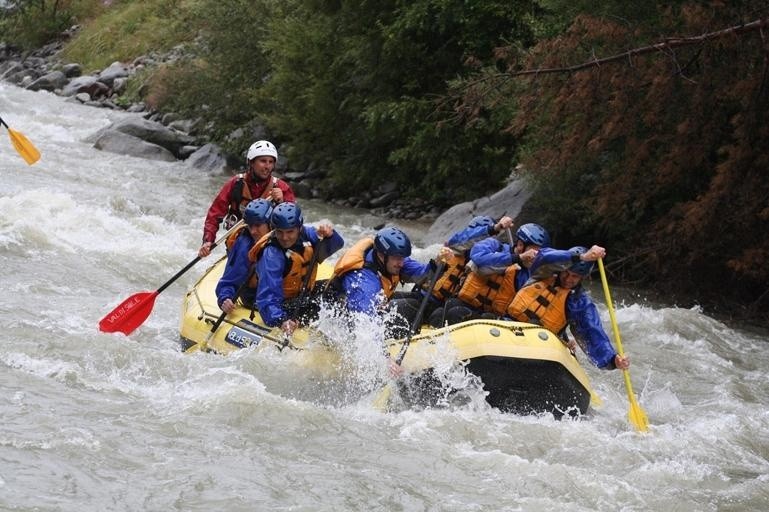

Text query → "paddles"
[100,193,272,336]
[185,261,256,357]
[0,119,42,166]
[371,250,450,415]
[592,252,648,432]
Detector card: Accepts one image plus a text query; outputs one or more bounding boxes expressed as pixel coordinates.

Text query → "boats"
[178,244,596,425]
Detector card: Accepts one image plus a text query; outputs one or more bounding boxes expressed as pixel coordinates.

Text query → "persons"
[479,243,630,369]
[427,220,578,353]
[411,214,514,324]
[322,227,454,384]
[197,140,297,259]
[247,201,345,336]
[214,198,276,315]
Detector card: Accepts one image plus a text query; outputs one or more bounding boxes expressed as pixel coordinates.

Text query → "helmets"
[242,198,276,225]
[270,201,303,229]
[567,246,595,277]
[468,215,496,228]
[373,226,412,258]
[246,139,279,166]
[515,223,552,248]
